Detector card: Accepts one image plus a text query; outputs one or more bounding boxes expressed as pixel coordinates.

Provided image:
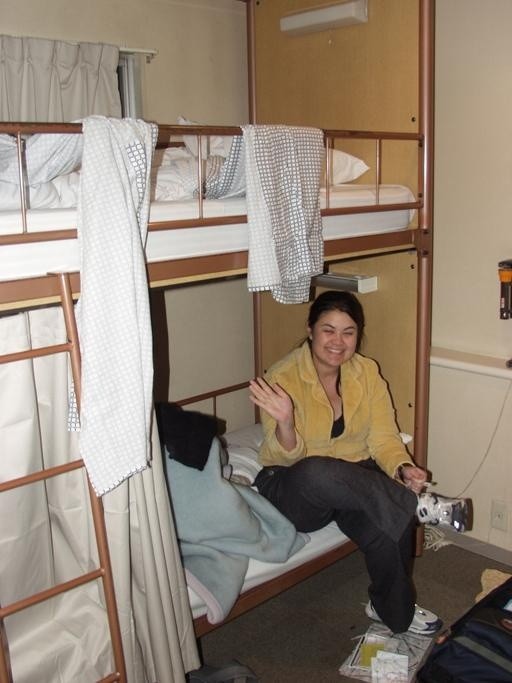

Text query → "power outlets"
[489,500,509,533]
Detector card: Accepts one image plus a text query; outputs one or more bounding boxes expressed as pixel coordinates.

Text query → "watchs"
[395,462,413,484]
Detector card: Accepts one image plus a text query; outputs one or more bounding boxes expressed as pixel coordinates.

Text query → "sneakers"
[364,599,442,635]
[413,478,469,536]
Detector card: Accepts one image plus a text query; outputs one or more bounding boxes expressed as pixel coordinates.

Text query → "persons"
[246,291,469,635]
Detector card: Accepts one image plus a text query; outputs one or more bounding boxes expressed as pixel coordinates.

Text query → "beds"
[0,378,430,683]
[1,114,423,314]
